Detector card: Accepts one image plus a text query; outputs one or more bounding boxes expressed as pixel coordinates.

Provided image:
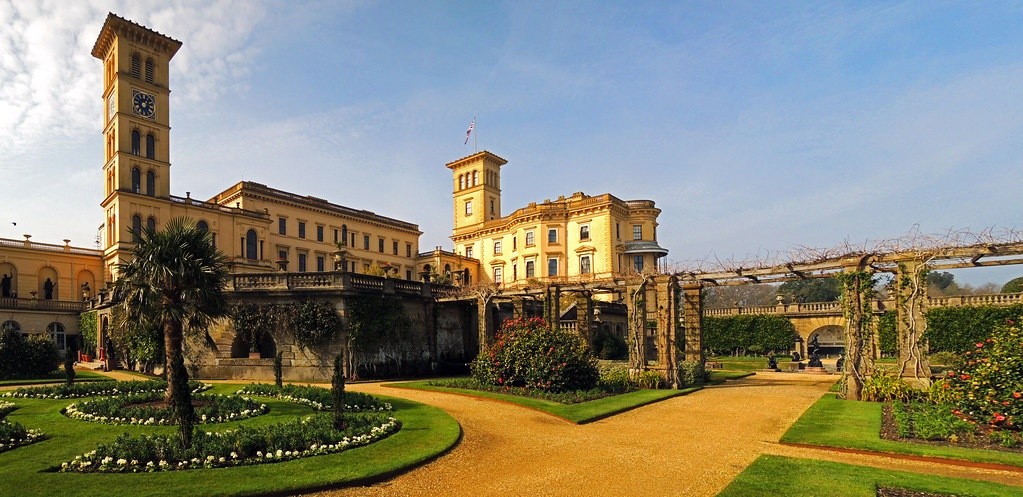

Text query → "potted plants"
[78,313,97,362]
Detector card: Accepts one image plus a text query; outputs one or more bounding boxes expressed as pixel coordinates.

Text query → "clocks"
[131,87,157,118]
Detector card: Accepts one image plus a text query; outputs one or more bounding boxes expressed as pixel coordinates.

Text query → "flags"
[465,120,474,143]
